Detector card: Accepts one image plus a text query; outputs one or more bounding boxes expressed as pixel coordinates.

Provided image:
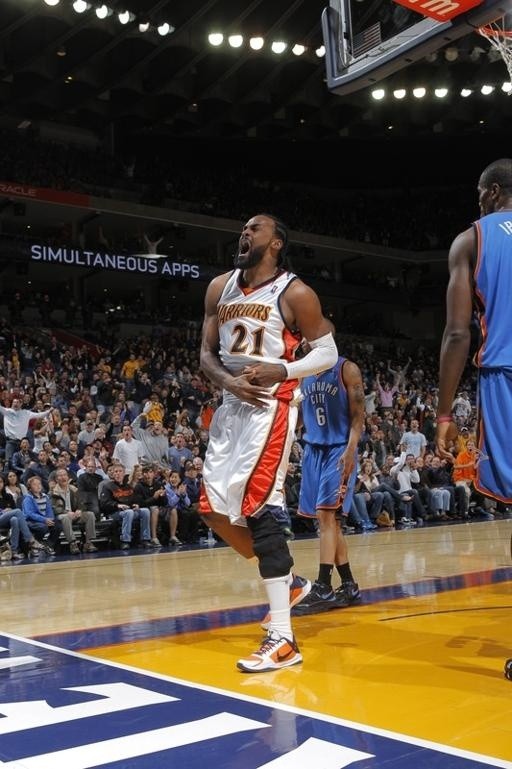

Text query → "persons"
[194,211,337,677]
[2,130,456,251]
[431,154,512,692]
[282,314,367,613]
[2,280,512,560]
[3,211,441,291]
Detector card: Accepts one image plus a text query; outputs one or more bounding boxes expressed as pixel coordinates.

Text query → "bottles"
[208,528,212,540]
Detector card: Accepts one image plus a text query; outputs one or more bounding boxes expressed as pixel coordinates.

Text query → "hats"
[460,427,468,432]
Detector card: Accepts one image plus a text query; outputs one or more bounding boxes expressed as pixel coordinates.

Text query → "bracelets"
[436,416,456,422]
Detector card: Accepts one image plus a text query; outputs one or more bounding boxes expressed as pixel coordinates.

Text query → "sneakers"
[259,572,312,631]
[342,506,507,532]
[292,580,362,610]
[236,629,303,673]
[10,536,217,560]
